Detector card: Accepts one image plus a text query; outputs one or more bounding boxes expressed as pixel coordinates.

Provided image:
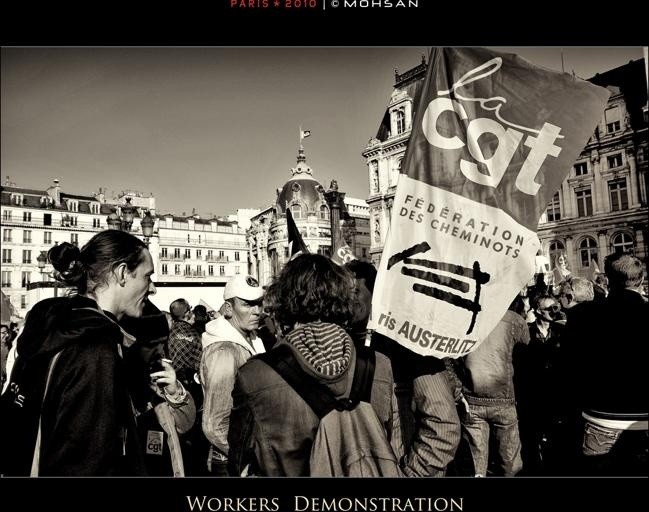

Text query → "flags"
[301,130,312,139]
[364,47,615,360]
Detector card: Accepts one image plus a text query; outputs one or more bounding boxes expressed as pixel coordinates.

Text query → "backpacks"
[262,344,402,478]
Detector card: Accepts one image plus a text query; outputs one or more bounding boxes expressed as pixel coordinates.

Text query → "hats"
[192,305,206,316]
[223,273,266,301]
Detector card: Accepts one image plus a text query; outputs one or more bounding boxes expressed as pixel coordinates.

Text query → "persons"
[0,229,647,476]
